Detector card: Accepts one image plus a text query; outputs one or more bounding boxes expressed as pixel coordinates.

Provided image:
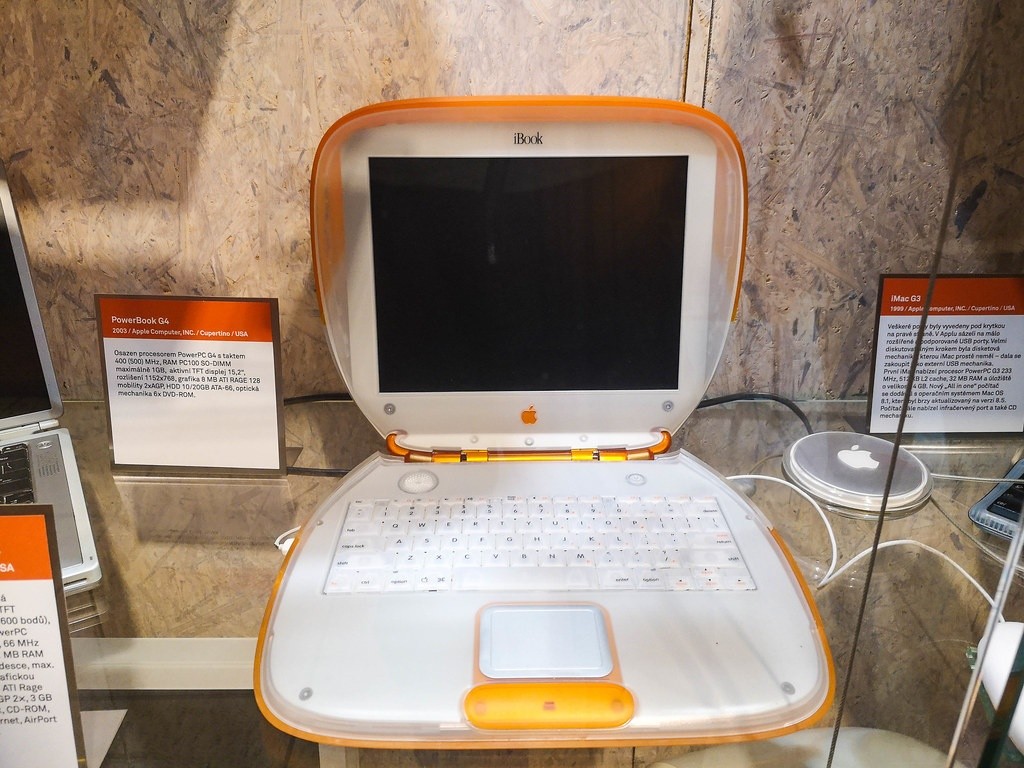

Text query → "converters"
[977,622,1024,755]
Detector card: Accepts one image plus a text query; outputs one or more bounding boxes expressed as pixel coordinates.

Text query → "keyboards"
[968,457,1024,543]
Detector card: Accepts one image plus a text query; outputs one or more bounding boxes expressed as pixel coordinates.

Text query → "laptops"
[251,96,837,746]
[0,158,103,592]
